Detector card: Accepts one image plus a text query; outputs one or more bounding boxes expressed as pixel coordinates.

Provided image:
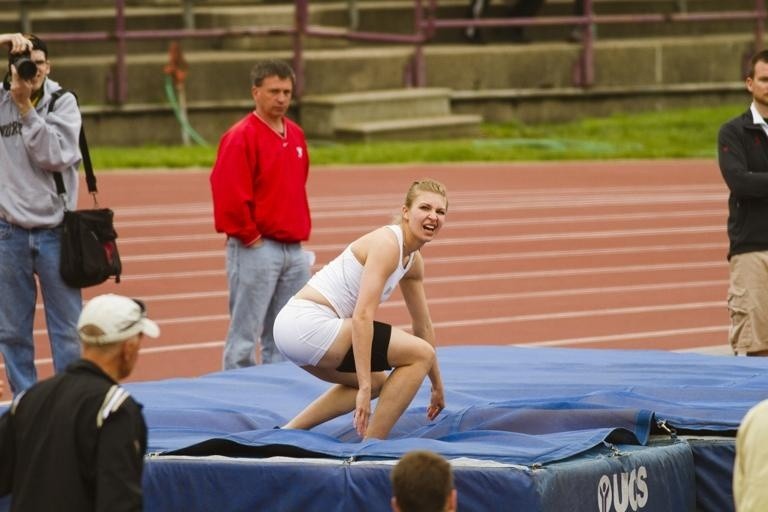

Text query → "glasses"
[118,299,145,333]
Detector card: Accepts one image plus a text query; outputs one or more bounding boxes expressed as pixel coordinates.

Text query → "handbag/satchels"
[62,209,122,287]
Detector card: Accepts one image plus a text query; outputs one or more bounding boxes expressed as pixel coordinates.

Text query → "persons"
[208,56,317,371]
[271,176,449,446]
[729,399,767,512]
[0,291,161,512]
[0,30,83,396]
[388,448,456,512]
[716,49,767,358]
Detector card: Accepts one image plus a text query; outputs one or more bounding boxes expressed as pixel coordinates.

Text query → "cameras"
[8,44,37,80]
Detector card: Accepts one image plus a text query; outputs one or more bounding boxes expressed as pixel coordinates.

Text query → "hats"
[76,293,160,343]
